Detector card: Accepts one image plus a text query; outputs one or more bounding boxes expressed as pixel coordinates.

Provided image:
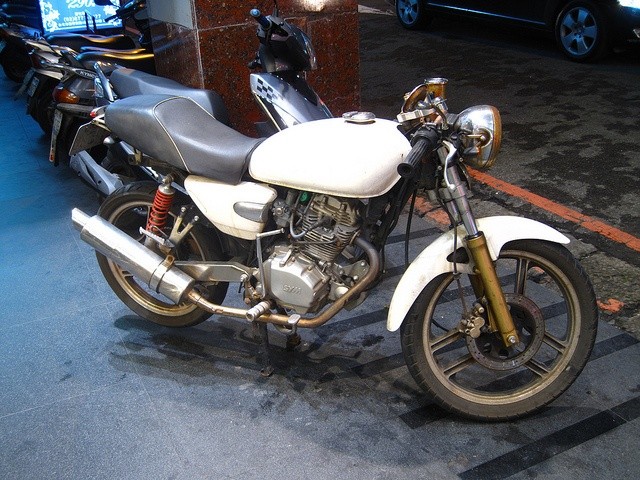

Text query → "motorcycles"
[151,18,195,82]
[0,0,154,81]
[68,76,599,423]
[41,50,154,168]
[67,1,339,208]
[18,36,151,135]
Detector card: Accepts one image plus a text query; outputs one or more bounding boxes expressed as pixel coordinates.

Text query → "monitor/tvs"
[35,0,129,43]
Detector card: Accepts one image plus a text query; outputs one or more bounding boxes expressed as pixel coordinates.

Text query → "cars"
[384,0,640,62]
